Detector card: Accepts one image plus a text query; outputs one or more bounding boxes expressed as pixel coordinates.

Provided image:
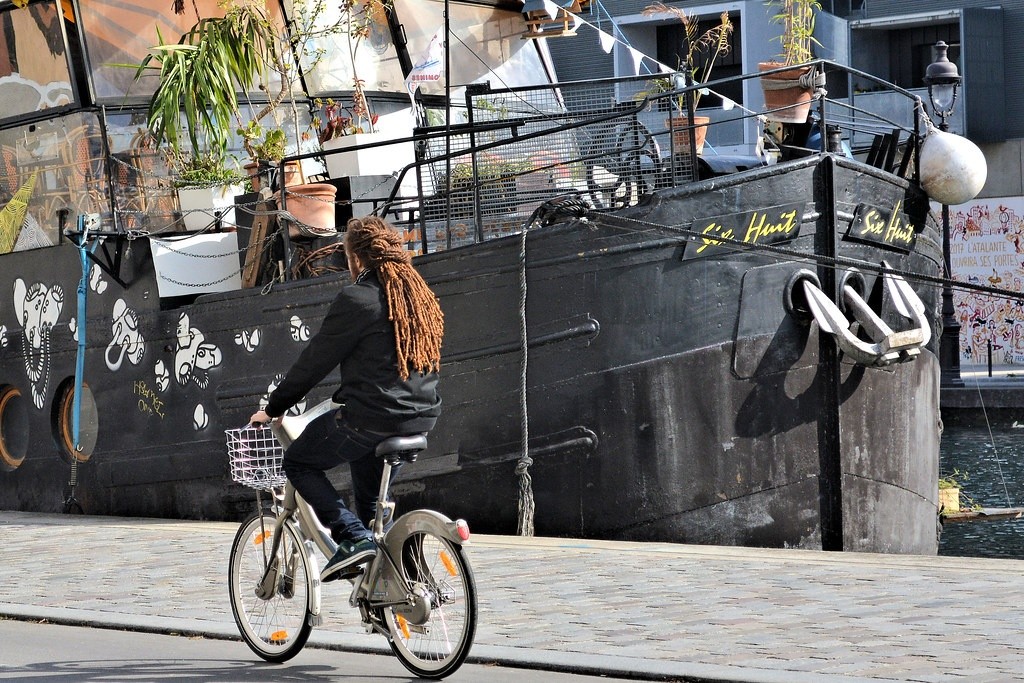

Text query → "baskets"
[224,427,287,491]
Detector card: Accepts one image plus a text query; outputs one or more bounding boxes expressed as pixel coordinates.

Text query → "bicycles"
[225,416,478,681]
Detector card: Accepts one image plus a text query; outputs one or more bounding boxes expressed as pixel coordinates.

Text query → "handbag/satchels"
[268,398,354,490]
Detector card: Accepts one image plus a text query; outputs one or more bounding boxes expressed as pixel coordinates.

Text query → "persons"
[250,215,444,634]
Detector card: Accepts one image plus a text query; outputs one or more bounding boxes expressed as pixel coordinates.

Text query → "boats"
[0,0,991,553]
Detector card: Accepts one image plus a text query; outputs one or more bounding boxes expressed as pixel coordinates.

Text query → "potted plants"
[757,0,821,126]
[221,0,394,196]
[632,2,733,156]
[322,0,395,178]
[234,3,337,239]
[436,95,552,215]
[122,5,275,231]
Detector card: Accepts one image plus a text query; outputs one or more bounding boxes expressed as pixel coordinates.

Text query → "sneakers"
[320,539,377,582]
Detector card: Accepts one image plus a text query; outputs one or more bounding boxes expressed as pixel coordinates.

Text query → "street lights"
[922,38,970,390]
